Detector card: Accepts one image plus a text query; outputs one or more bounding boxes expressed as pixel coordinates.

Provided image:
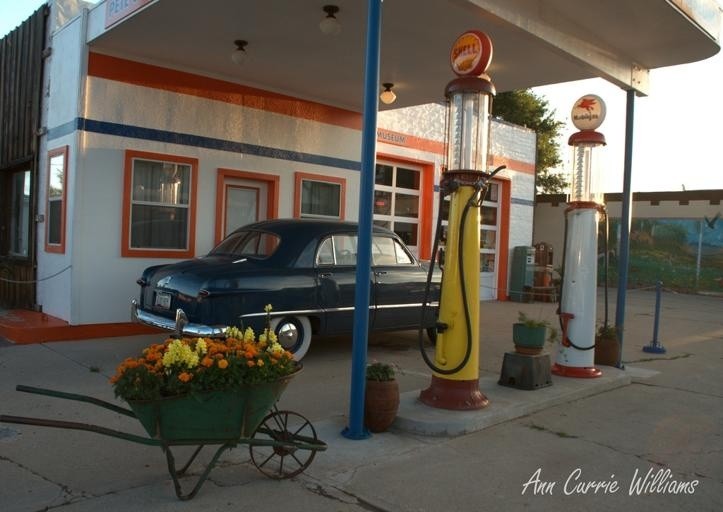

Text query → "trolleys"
[0,360,326,502]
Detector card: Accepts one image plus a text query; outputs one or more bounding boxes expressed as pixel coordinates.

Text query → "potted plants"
[595,320,626,367]
[364,361,400,434]
[512,310,557,355]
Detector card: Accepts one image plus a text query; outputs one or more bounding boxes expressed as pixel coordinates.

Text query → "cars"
[126,216,444,367]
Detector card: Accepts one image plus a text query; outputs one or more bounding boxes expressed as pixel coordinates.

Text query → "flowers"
[107,305,299,403]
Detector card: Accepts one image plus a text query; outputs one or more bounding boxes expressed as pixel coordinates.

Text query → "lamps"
[230,40,247,65]
[318,5,339,35]
[380,83,396,105]
